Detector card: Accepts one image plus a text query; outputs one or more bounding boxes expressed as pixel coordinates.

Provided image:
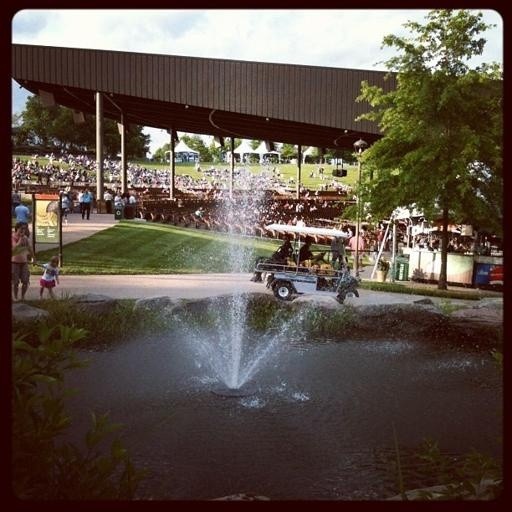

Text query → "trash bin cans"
[476,263,490,284]
[115,203,123,220]
[396,253,409,281]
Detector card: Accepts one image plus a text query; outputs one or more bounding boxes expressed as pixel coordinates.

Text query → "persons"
[250,234,293,282]
[332,227,367,272]
[34,256,60,299]
[298,234,331,290]
[15,201,30,238]
[12,222,31,301]
[11,149,355,220]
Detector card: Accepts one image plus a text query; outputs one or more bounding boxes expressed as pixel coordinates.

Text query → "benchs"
[20,179,353,242]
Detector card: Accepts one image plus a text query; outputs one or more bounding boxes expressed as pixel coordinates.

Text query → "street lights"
[350,136,368,281]
